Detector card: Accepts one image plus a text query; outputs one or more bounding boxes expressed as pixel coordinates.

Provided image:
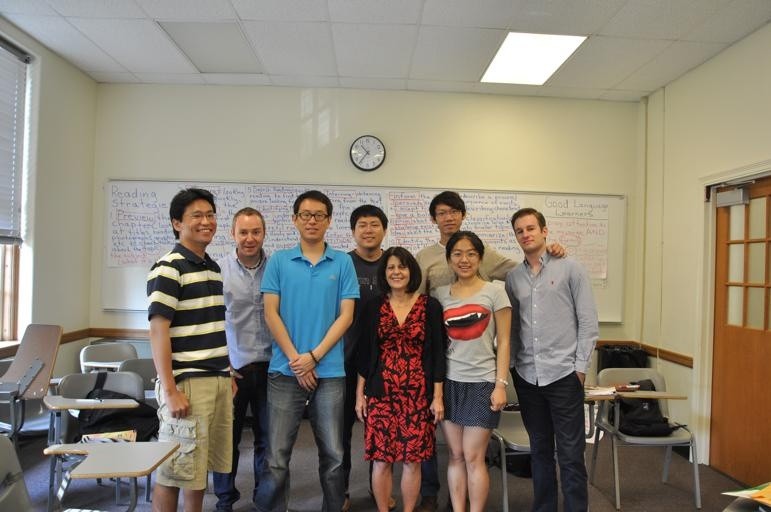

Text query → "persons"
[146,188,244,512]
[354,246,446,512]
[253,190,361,512]
[214,207,273,512]
[505,208,600,512]
[432,231,514,512]
[343,205,398,511]
[414,190,567,512]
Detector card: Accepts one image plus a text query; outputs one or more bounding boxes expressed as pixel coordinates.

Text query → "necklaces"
[244,255,262,271]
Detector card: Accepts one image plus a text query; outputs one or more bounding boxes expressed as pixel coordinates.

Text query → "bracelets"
[496,376,509,387]
[309,350,319,364]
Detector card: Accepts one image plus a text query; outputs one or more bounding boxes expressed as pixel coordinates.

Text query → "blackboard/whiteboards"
[101,179,626,324]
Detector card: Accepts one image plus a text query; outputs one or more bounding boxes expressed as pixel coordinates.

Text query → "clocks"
[351,135,388,172]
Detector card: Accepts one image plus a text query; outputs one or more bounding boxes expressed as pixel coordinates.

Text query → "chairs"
[0,318,184,511]
[480,367,702,511]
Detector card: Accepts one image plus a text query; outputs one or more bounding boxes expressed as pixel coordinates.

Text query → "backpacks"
[609,380,688,436]
[75,371,158,441]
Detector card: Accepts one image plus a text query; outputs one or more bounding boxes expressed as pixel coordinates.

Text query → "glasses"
[296,211,329,222]
